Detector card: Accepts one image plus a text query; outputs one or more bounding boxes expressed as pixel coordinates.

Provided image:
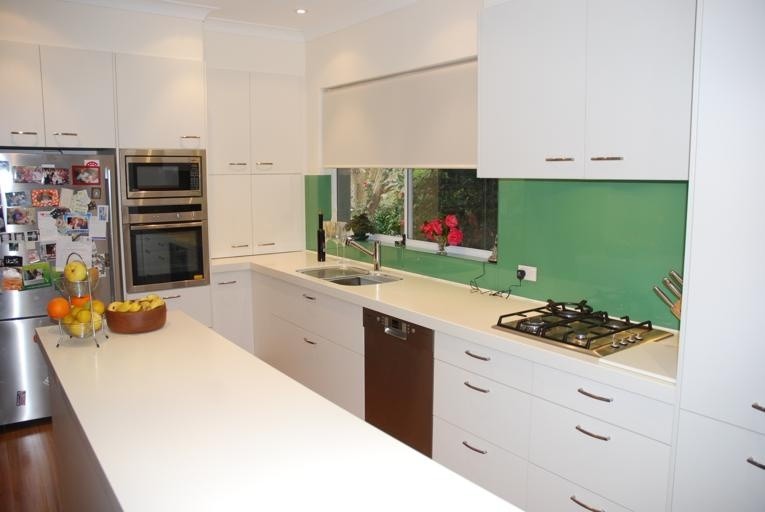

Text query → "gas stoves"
[491,296,674,360]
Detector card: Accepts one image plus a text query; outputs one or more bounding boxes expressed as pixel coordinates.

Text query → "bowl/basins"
[60,275,99,298]
[58,318,103,339]
[105,300,166,335]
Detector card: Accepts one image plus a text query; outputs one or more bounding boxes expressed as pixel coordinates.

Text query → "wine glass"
[328,222,350,266]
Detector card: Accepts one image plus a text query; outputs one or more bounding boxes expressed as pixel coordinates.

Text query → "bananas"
[108,294,162,312]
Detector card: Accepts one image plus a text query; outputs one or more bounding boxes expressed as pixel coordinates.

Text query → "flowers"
[419,214,464,245]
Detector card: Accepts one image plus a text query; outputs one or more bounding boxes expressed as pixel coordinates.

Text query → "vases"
[435,237,449,253]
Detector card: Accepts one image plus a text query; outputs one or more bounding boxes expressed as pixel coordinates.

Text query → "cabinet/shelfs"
[525,342,675,512]
[114,51,207,151]
[206,174,306,258]
[207,66,308,174]
[476,0,696,181]
[251,271,366,421]
[0,39,117,149]
[123,283,212,326]
[431,329,534,512]
[209,269,254,355]
[669,0,765,512]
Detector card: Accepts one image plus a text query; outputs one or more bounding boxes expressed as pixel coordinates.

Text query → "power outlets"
[516,264,537,282]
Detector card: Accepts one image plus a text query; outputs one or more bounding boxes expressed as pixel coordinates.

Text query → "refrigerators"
[1,147,123,426]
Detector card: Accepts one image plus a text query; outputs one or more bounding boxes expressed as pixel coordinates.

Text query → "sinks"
[320,272,404,286]
[295,263,370,280]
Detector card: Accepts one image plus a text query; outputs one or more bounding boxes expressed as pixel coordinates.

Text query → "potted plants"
[344,213,376,237]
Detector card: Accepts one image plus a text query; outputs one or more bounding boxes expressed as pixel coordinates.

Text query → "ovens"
[117,146,209,296]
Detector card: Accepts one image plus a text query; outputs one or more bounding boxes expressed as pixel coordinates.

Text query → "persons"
[77,169,98,183]
[46,169,67,184]
[69,218,88,229]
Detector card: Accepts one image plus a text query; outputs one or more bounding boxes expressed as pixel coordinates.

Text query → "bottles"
[316,213,326,263]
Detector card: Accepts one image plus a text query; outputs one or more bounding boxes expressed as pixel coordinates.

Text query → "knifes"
[668,270,683,288]
[653,286,675,309]
[662,278,681,300]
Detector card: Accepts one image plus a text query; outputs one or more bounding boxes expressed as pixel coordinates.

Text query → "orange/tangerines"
[62,300,104,336]
[66,262,85,282]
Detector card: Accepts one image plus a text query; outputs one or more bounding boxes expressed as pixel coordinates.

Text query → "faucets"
[345,238,382,271]
